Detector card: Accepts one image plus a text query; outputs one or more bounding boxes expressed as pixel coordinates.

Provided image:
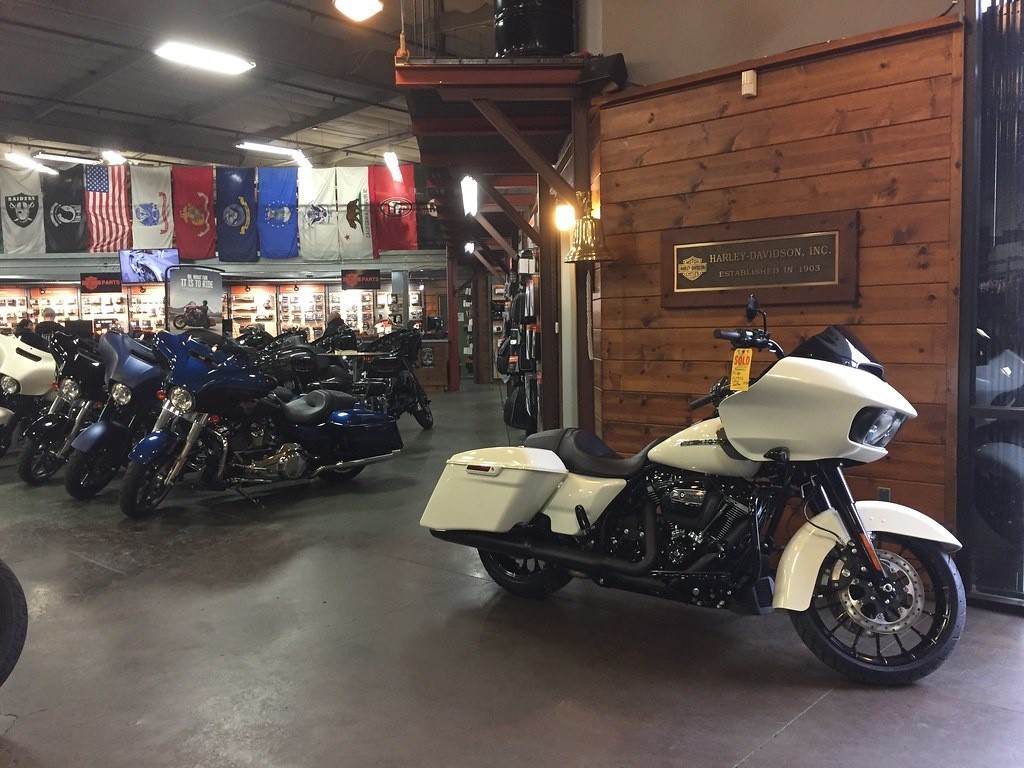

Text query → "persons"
[17,319,33,331]
[35,308,65,333]
[197,300,209,325]
[322,311,344,336]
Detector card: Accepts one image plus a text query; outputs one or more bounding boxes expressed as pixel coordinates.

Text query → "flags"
[0,158,46,254]
[373,164,418,253]
[172,166,216,260]
[259,166,298,261]
[39,164,89,253]
[337,166,380,261]
[297,168,340,261]
[414,164,453,249]
[130,166,174,249]
[216,167,258,262]
[84,165,131,253]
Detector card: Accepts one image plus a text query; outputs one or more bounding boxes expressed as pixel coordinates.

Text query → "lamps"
[459,172,478,217]
[5,138,127,175]
[553,195,575,230]
[234,131,313,169]
[384,120,402,182]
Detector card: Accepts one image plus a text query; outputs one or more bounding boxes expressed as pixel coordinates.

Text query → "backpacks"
[509,292,522,324]
[503,384,527,430]
[496,335,511,374]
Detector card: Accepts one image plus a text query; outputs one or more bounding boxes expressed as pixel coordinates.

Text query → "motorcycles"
[0,318,433,518]
[173,306,216,330]
[973,327,1024,572]
[419,294,967,682]
